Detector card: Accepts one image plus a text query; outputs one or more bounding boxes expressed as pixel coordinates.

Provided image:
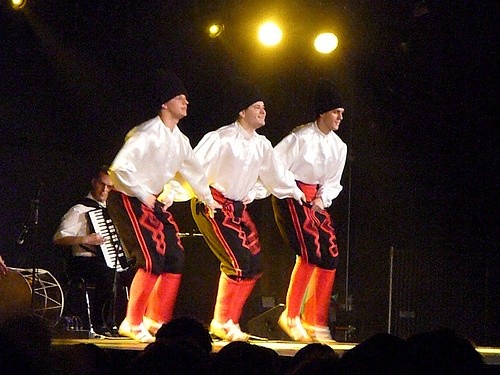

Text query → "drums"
[0,265,65,331]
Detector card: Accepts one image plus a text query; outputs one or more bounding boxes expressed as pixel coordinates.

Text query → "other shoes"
[91,316,111,336]
[104,320,119,336]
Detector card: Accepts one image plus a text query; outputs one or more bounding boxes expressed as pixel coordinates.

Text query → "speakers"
[247,303,292,341]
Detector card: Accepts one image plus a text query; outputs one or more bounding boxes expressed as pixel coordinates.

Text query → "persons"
[158,77,306,343]
[243,80,347,344]
[52,165,135,337]
[107,64,223,343]
[0,257,500,375]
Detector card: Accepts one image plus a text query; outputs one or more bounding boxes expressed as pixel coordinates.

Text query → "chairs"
[49,244,99,339]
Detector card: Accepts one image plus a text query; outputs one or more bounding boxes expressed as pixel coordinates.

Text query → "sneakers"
[209,318,249,342]
[277,309,312,343]
[143,316,162,335]
[118,318,156,343]
[309,324,336,342]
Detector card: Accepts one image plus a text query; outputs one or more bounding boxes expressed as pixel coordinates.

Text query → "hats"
[232,77,264,115]
[155,71,188,108]
[315,79,340,115]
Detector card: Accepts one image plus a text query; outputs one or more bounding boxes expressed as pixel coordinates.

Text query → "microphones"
[17,225,29,244]
